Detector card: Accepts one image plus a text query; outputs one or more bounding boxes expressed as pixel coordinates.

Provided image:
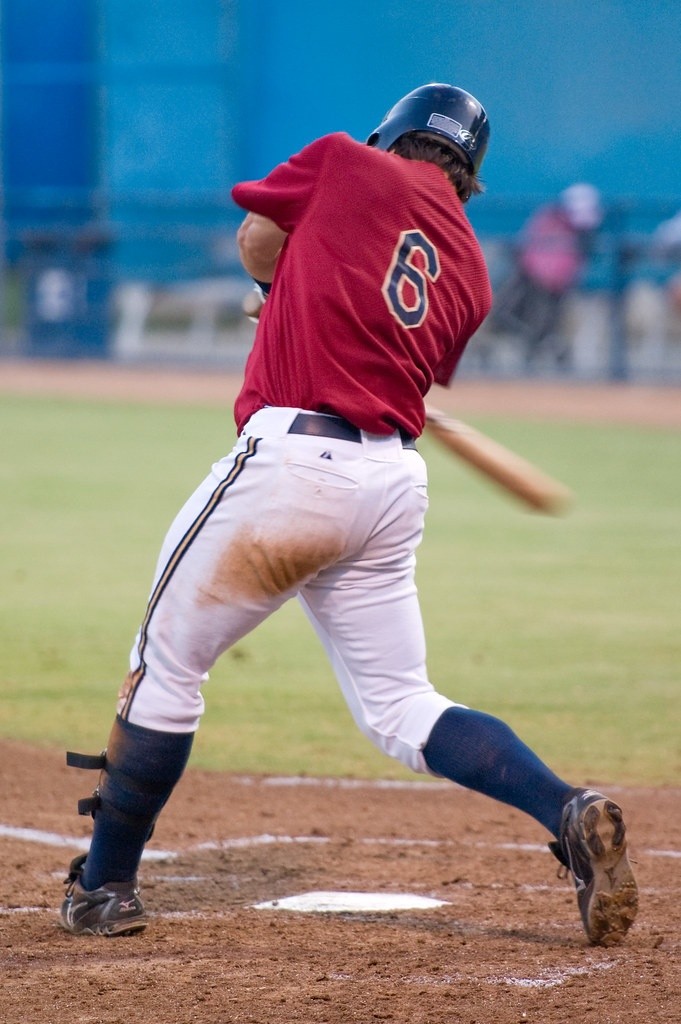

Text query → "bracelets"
[251,278,272,298]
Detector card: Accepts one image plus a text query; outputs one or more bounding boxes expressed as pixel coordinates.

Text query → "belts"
[243,414,419,452]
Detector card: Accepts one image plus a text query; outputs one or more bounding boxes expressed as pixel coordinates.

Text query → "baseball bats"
[241,292,559,513]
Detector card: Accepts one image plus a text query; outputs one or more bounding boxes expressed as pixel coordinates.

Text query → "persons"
[487,185,681,378]
[56,82,638,944]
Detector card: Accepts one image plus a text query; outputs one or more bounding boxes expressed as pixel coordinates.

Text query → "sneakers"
[548,787,639,949]
[59,853,149,936]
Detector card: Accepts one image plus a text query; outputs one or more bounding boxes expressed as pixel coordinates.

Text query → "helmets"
[366,83,490,204]
